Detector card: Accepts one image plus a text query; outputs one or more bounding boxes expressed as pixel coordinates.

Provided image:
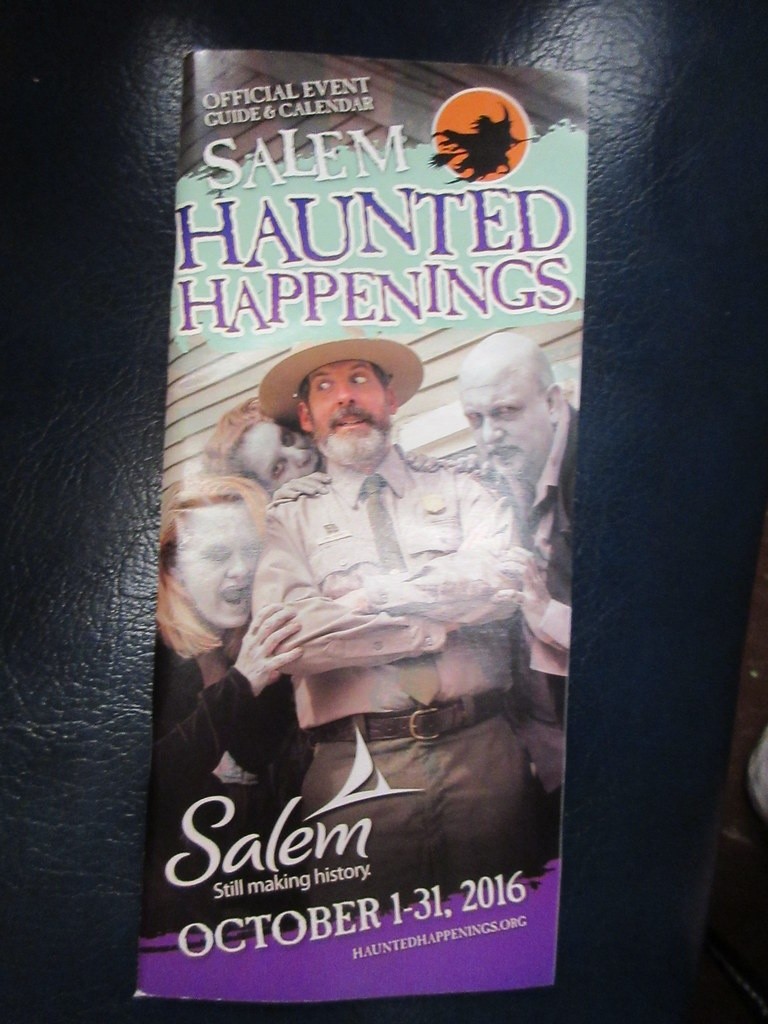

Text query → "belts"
[314,690,505,743]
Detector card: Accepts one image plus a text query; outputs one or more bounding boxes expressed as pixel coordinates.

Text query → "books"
[135,49,588,1000]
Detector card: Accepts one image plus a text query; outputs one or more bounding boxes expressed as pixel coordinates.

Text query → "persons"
[149,332,578,937]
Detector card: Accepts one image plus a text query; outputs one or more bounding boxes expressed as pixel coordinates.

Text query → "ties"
[518,487,557,553]
[359,475,440,705]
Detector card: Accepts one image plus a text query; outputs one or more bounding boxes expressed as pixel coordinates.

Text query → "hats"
[258,326,424,422]
